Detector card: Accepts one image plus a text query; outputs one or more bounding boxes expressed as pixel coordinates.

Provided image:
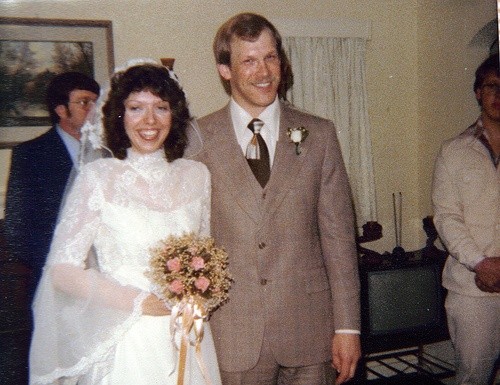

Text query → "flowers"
[286,126,309,155]
[143,230,235,319]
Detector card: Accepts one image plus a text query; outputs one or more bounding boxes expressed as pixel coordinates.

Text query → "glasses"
[476,82,500,93]
[62,98,99,108]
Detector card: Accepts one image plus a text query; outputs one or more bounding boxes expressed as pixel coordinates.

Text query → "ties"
[245,118,272,189]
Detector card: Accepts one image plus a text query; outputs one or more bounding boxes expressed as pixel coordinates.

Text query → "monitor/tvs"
[356,256,444,350]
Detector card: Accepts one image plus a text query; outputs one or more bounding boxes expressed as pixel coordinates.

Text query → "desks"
[359,328,457,385]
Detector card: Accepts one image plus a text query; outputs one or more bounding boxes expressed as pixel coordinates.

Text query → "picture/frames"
[0,17,115,149]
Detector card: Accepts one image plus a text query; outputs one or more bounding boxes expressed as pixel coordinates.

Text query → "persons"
[432,56,500,385]
[4,71,100,385]
[27,58,214,385]
[183,12,362,385]
